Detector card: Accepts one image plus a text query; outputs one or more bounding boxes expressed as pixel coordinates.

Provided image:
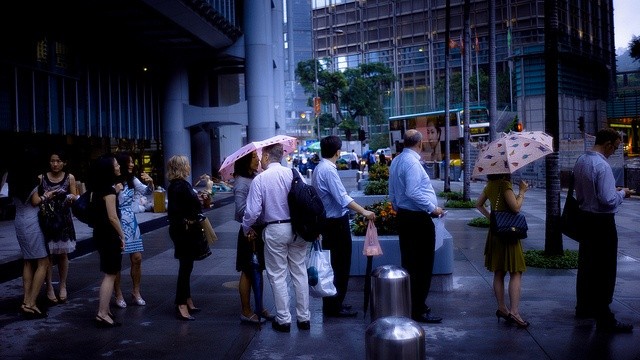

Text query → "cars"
[340,153,351,161]
[374,148,392,164]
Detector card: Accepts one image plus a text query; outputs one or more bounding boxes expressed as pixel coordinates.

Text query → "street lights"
[327,29,344,137]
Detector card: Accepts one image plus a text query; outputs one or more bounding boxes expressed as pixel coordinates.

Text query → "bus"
[389,106,491,167]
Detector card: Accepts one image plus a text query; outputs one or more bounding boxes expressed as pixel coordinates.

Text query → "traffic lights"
[514,123,522,132]
[577,116,585,132]
[358,128,367,140]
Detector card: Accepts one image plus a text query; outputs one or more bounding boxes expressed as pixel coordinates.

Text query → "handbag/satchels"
[305,240,337,298]
[37,196,66,233]
[72,191,95,228]
[288,169,326,242]
[562,191,580,243]
[199,215,219,246]
[490,192,528,239]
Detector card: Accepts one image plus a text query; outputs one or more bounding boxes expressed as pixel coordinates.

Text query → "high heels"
[95,311,122,328]
[496,310,508,324]
[508,313,529,326]
[20,303,48,319]
[176,304,202,321]
[132,294,146,305]
[114,297,127,308]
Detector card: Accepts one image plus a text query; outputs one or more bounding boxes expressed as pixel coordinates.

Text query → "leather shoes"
[297,319,310,330]
[595,321,633,329]
[272,318,290,333]
[324,309,357,317]
[343,304,352,309]
[411,307,442,323]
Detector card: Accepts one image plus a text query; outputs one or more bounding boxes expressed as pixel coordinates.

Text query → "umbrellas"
[473,130,555,179]
[362,215,374,318]
[218,134,298,181]
[248,228,263,332]
[307,141,321,152]
[361,150,373,159]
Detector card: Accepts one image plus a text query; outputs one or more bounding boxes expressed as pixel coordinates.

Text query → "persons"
[233,149,275,323]
[573,127,636,333]
[379,150,386,164]
[11,155,57,319]
[427,117,442,160]
[367,153,375,172]
[242,143,323,332]
[311,135,376,319]
[92,153,126,328]
[166,154,204,322]
[350,150,359,169]
[388,129,444,323]
[114,151,156,308]
[475,174,530,329]
[38,152,81,306]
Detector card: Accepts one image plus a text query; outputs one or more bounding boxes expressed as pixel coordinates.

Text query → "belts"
[269,220,291,224]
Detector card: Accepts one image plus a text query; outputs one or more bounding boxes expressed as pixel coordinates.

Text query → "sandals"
[252,309,276,320]
[240,314,266,323]
